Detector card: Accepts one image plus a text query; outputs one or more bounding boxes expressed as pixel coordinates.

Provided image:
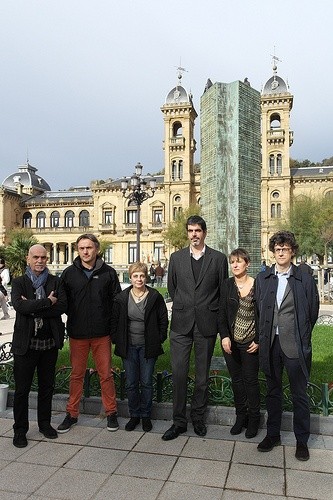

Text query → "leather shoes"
[295,440,309,461]
[257,435,281,452]
[162,424,187,441]
[192,422,207,436]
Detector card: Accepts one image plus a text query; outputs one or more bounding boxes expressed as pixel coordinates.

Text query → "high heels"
[230,414,248,435]
[245,415,260,439]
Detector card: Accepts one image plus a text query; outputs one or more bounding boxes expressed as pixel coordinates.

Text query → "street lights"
[120,162,156,262]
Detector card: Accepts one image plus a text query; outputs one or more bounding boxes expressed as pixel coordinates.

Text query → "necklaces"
[132,287,146,301]
[238,286,243,289]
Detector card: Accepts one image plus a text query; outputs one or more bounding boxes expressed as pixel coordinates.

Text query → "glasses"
[274,248,293,252]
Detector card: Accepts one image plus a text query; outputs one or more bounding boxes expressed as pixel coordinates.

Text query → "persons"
[55,234,123,434]
[218,248,258,440]
[161,215,229,440]
[0,275,8,336]
[261,261,266,272]
[253,231,320,461]
[111,262,169,432]
[155,262,165,287]
[10,244,68,448]
[150,264,156,287]
[0,258,10,320]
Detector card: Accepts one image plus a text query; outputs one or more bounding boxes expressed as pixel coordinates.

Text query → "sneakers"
[39,425,58,439]
[13,428,28,448]
[142,417,153,432]
[56,412,78,433]
[125,417,140,431]
[106,412,120,431]
